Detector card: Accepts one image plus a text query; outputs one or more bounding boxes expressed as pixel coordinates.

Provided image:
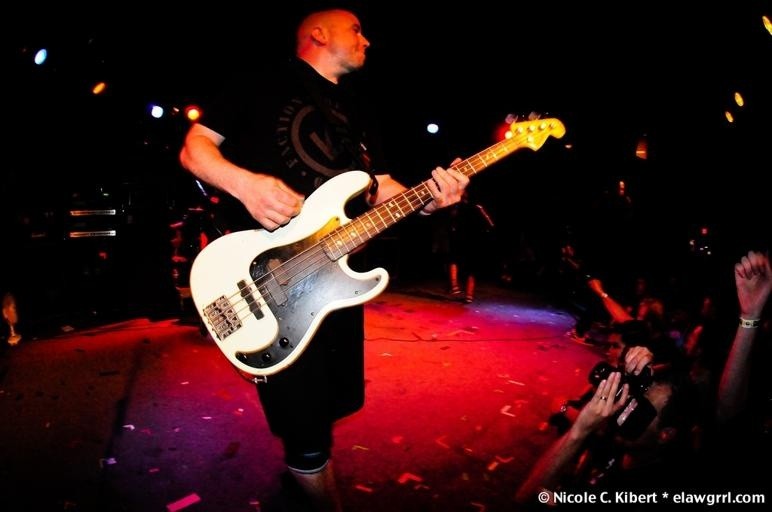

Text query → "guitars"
[189,113,567,384]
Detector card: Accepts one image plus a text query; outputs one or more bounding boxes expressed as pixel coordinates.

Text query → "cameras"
[587,360,657,444]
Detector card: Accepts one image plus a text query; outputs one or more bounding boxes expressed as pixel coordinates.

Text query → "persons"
[178,7,472,511]
[442,188,479,302]
[507,176,772,511]
